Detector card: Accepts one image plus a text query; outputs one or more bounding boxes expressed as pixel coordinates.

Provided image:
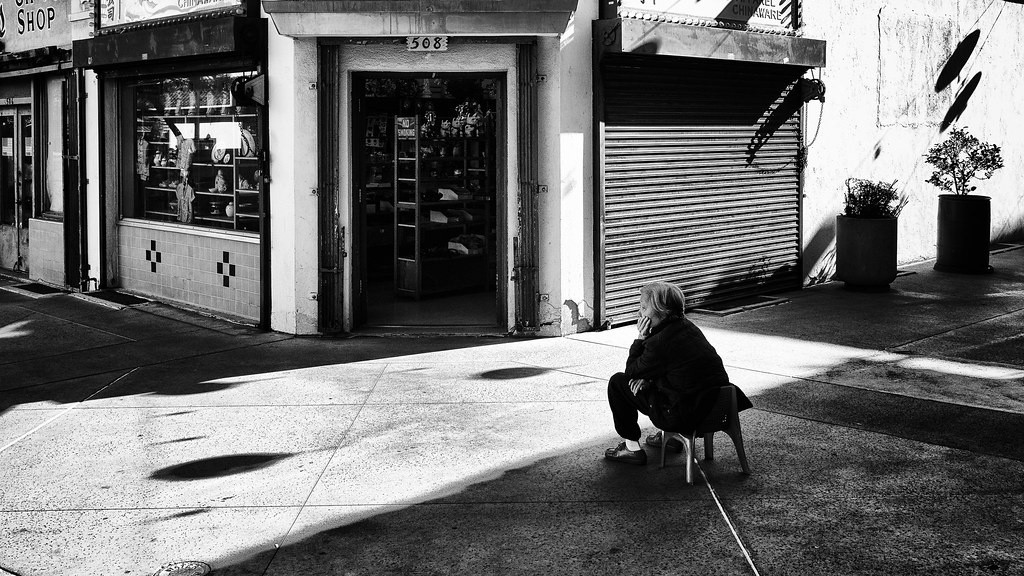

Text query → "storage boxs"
[429,208,462,223]
[437,188,472,201]
[457,210,481,221]
[447,235,483,255]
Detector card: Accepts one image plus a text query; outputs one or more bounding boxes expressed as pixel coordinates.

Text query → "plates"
[210,143,225,164]
[223,154,230,163]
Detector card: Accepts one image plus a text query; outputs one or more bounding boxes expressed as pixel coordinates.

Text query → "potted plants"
[836,176,910,292]
[925,123,1004,272]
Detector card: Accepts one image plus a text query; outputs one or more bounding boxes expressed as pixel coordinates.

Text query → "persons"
[605,281,753,466]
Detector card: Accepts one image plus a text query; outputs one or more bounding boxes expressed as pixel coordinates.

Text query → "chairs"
[661,385,751,483]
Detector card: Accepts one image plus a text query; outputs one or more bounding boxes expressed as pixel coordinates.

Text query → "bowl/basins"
[209,188,218,193]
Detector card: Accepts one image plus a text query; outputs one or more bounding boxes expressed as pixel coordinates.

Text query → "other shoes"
[605,442,647,465]
[646,430,683,449]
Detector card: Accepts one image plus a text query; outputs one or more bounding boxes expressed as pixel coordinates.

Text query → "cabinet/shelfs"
[139,113,262,234]
[356,112,494,297]
